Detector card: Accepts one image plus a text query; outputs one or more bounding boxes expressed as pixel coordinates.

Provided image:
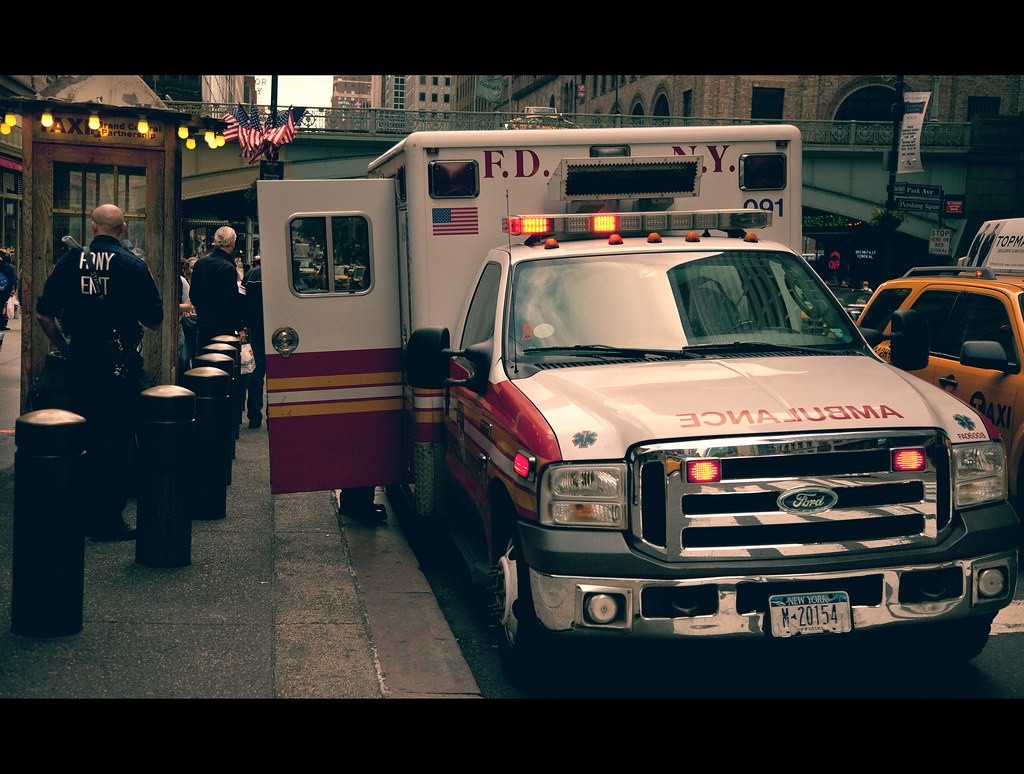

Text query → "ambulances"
[255,123,1024,691]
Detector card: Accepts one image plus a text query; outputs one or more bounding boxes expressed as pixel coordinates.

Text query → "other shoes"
[249,418,261,428]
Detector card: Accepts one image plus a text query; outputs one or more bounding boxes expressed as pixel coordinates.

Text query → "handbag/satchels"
[111,348,144,397]
[240,337,256,375]
[5,296,20,319]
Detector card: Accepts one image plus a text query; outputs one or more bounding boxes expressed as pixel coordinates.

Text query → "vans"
[957,219,1024,276]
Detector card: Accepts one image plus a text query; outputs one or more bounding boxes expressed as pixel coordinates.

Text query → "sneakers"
[340,493,387,522]
[88,522,137,543]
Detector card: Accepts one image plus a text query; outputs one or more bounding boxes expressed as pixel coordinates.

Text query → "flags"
[222,103,307,164]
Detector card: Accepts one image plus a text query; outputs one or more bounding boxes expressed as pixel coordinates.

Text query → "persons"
[37,204,167,540]
[861,281,873,292]
[339,487,388,522]
[180,225,269,428]
[841,281,848,287]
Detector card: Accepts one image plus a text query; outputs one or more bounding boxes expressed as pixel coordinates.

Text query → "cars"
[826,286,873,319]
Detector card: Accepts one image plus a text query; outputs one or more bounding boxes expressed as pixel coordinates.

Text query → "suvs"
[795,264,1024,518]
